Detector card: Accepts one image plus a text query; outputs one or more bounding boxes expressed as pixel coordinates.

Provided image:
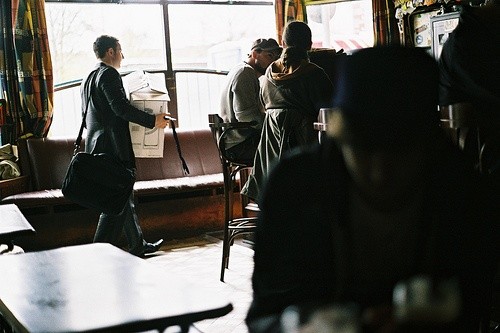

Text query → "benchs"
[0,129,241,249]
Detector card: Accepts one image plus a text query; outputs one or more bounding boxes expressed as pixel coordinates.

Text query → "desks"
[0,242,232,333]
[0,203,36,252]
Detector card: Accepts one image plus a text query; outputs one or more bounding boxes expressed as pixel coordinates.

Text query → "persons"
[221,39,282,167]
[245,42,500,333]
[79,34,171,257]
[436,0,500,169]
[240,19,338,202]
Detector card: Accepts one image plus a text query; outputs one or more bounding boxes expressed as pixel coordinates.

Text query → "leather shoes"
[129,238,164,258]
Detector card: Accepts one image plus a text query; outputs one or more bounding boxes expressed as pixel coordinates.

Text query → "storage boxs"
[123,70,171,159]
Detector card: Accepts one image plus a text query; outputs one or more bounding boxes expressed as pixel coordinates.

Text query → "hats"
[330,46,440,114]
[251,38,283,51]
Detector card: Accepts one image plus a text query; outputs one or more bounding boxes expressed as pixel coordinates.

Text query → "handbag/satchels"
[62,152,136,216]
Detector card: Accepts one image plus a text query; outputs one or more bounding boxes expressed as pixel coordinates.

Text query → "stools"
[208,114,260,281]
